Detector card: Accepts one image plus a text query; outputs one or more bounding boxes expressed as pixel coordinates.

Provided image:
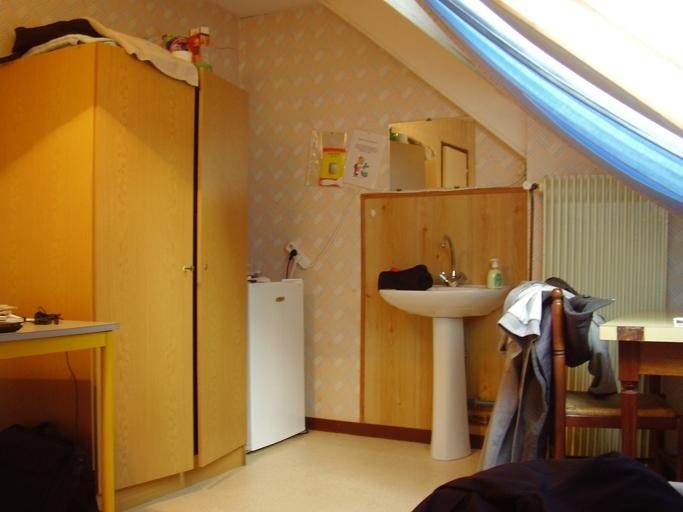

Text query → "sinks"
[378,284,507,317]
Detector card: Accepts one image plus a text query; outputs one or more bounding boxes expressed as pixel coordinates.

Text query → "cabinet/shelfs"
[0,41,248,512]
[390,133,429,190]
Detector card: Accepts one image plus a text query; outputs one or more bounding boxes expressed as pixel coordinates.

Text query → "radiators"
[540,173,669,459]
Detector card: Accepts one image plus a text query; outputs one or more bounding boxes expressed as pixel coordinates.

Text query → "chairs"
[540,288,677,477]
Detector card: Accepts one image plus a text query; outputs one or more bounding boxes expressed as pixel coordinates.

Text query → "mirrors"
[389,118,526,189]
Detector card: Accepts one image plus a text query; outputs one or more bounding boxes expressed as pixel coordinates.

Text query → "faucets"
[438,234,467,287]
